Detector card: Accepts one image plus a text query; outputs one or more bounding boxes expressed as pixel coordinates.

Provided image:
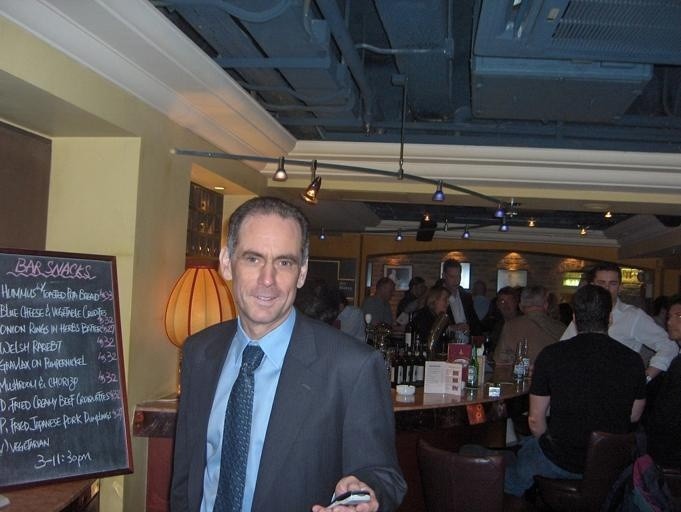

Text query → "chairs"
[529,417,648,512]
[403,433,510,511]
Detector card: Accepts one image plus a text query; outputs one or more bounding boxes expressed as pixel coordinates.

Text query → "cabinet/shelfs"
[0,467,105,512]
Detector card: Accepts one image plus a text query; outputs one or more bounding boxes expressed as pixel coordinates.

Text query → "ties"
[213,345,265,512]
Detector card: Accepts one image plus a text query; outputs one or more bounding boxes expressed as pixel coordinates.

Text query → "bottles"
[466,343,479,388]
[513,338,532,381]
[390,335,431,389]
[485,340,496,383]
[404,312,416,350]
[466,390,478,401]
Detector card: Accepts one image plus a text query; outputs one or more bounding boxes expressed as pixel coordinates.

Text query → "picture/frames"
[495,266,528,296]
[438,260,472,292]
[381,262,413,292]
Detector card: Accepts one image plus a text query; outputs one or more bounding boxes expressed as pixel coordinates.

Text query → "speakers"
[417,219,436,242]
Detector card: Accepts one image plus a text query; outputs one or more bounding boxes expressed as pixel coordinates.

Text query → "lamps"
[162,253,238,401]
[261,157,515,241]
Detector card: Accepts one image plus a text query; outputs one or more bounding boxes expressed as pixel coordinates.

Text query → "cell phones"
[325,491,370,510]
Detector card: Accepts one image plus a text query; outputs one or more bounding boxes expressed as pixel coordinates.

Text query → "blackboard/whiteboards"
[0,248,133,492]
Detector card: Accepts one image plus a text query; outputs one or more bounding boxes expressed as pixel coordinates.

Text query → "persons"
[169,197,408,512]
